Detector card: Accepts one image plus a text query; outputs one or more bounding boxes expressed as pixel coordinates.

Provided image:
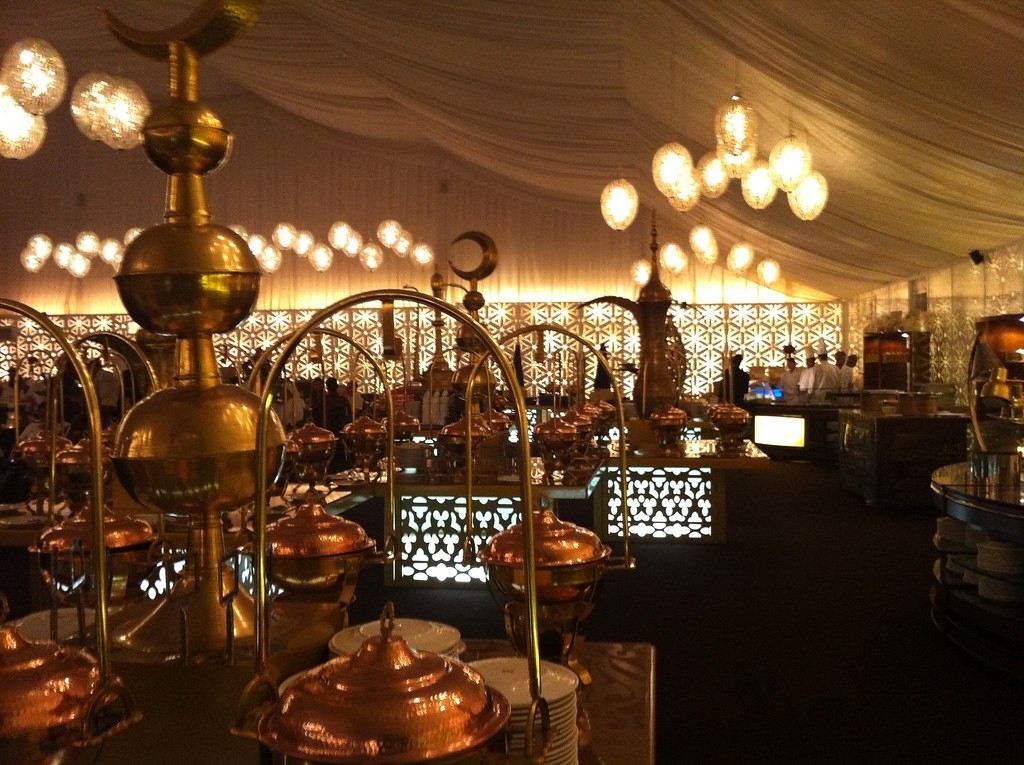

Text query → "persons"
[718,355,750,407]
[0,358,364,470]
[780,351,861,397]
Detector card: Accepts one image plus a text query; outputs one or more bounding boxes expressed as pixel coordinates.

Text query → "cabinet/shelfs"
[863,332,932,395]
[930,462,1024,681]
[838,408,973,517]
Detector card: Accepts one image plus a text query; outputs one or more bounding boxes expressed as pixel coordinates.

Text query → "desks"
[0,487,374,553]
[3,605,349,765]
[592,418,769,544]
[374,408,608,589]
[459,638,656,765]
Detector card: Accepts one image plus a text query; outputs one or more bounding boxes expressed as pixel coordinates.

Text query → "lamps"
[600,56,830,231]
[1,38,67,159]
[70,71,153,150]
[979,368,1011,408]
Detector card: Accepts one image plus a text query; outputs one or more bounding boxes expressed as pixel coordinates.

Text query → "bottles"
[422,390,430,424]
[441,391,450,424]
[431,391,440,424]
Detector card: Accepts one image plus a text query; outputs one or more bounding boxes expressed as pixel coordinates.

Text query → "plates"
[396,445,427,470]
[407,401,421,416]
[466,657,579,765]
[329,618,466,659]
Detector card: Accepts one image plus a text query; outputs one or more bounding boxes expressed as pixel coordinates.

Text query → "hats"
[818,340,828,356]
[805,346,815,359]
